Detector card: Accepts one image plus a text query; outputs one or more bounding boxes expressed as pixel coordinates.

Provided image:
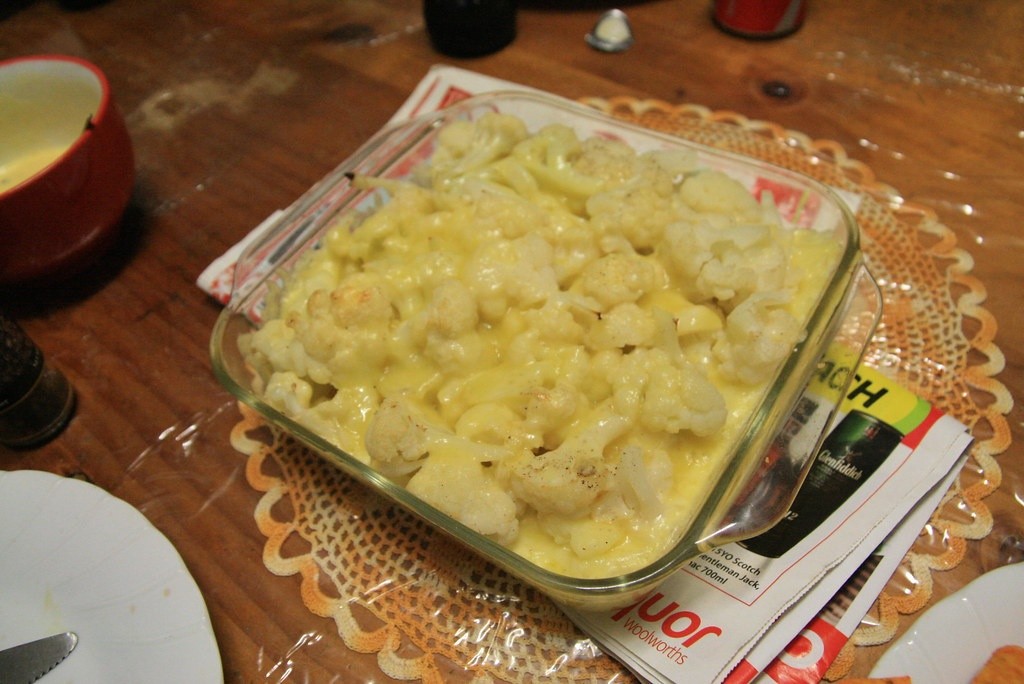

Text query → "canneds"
[735,409,904,558]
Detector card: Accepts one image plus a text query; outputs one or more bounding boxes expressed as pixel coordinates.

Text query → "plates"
[865,561,1024,684]
[0,469,224,684]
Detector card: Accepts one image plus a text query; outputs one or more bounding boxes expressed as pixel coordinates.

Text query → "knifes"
[0,631,79,684]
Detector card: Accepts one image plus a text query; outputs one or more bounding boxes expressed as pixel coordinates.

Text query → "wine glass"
[979,418,1024,573]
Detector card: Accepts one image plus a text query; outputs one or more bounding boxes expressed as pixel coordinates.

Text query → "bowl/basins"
[0,54,136,284]
[209,90,884,614]
